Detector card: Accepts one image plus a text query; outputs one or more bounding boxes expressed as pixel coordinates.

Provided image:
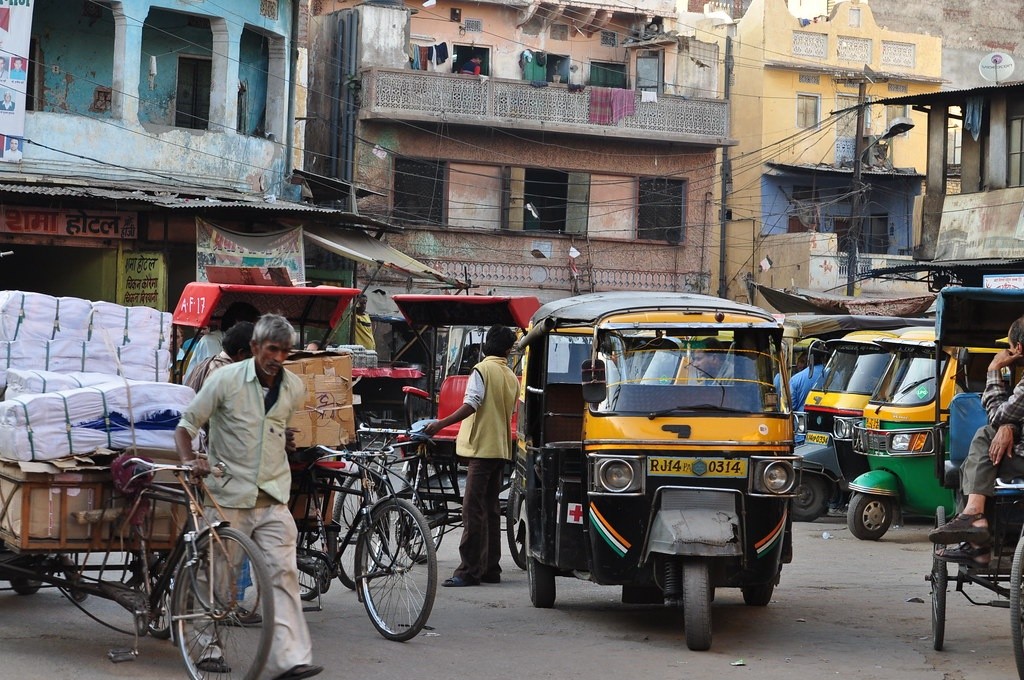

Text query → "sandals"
[928,513,990,544]
[933,542,991,569]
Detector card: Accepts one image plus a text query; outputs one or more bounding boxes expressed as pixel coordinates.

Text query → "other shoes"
[214,607,262,628]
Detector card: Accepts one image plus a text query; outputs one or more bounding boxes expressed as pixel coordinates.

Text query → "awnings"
[280,218,479,288]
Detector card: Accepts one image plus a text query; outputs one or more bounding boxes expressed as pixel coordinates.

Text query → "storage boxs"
[281,355,358,449]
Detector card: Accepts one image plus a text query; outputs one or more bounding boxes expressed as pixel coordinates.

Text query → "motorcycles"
[632,310,1010,541]
[518,291,804,654]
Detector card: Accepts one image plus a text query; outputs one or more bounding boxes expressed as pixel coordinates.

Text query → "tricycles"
[0,454,276,680]
[168,265,542,642]
[923,285,1024,680]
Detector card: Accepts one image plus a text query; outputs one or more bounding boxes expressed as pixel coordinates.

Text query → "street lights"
[847,115,917,297]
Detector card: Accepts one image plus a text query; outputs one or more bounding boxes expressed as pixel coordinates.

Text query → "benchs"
[402,375,521,439]
[943,392,987,488]
[537,382,755,488]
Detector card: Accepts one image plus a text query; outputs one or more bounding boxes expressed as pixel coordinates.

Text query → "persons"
[182,302,260,385]
[774,351,843,446]
[354,294,377,352]
[422,324,520,588]
[183,324,210,351]
[927,316,1024,569]
[174,313,325,680]
[185,320,255,433]
[169,327,193,385]
[692,338,734,386]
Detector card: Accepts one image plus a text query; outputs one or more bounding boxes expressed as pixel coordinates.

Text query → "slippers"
[441,576,480,586]
[196,656,232,673]
[275,664,324,680]
[480,577,501,584]
